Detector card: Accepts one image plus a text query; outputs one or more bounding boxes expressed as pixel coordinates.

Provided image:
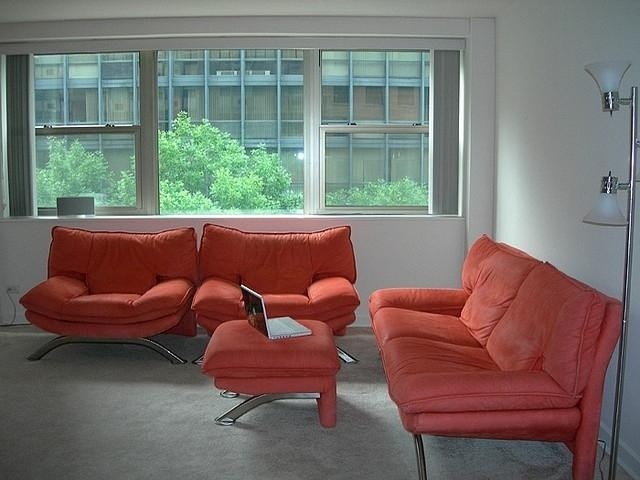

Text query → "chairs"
[190,223,359,364]
[20,224,198,364]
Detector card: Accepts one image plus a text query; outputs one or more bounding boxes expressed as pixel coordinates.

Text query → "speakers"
[56,197,95,218]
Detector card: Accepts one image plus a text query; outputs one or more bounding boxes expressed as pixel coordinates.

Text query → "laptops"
[241,284,312,339]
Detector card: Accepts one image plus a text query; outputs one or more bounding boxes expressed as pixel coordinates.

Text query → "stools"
[203,318,339,428]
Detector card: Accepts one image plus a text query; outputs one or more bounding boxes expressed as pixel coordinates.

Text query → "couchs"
[366,233,624,479]
